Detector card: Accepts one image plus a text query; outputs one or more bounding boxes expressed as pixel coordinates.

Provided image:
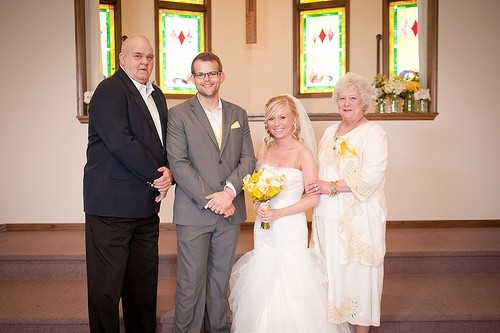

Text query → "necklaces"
[335,115,364,141]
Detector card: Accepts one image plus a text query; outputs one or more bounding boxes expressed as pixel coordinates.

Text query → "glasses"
[192,71,221,79]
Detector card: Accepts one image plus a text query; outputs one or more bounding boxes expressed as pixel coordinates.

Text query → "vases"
[376,95,385,113]
[386,94,400,113]
[420,99,429,113]
[405,92,414,113]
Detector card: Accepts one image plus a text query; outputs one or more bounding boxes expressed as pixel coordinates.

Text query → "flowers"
[368,70,433,104]
[241,163,288,229]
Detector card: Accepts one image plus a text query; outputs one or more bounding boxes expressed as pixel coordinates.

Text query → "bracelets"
[328,180,340,196]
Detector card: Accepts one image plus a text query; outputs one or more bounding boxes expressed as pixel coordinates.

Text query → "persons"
[84,34,172,333]
[306,72,389,332]
[166,51,256,332]
[248,95,318,333]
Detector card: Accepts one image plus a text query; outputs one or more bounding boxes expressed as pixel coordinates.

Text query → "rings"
[314,183,317,187]
[315,188,319,190]
